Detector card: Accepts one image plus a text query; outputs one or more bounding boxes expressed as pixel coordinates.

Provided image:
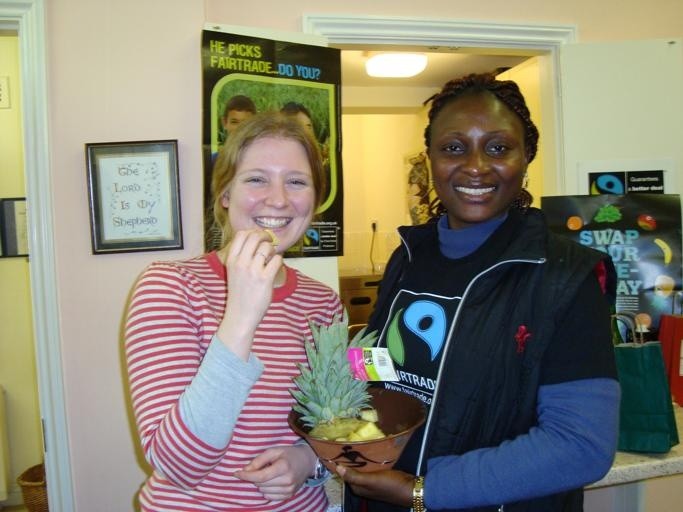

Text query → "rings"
[254,250,267,261]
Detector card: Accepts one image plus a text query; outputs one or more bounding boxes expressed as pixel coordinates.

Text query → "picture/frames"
[0,196,28,258]
[84,139,183,254]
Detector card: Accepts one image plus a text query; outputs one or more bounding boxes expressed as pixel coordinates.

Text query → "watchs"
[311,450,327,482]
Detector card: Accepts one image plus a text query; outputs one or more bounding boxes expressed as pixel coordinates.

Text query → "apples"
[638,214,656,231]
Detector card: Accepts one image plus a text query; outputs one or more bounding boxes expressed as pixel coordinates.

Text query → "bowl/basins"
[286,385,429,477]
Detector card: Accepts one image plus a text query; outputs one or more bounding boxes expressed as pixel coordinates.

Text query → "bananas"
[654,239,673,264]
[594,203,621,223]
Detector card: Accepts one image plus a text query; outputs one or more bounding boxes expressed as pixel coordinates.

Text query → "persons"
[207,93,259,174]
[276,101,317,144]
[123,110,350,511]
[318,70,624,511]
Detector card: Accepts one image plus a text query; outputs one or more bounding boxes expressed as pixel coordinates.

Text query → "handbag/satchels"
[657,314,683,411]
[614,342,678,455]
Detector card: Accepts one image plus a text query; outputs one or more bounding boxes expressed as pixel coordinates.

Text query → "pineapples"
[308,407,386,442]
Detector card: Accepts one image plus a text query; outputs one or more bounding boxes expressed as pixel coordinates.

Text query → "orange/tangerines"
[567,216,582,230]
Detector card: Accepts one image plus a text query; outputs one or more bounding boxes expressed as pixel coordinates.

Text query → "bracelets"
[409,473,426,512]
